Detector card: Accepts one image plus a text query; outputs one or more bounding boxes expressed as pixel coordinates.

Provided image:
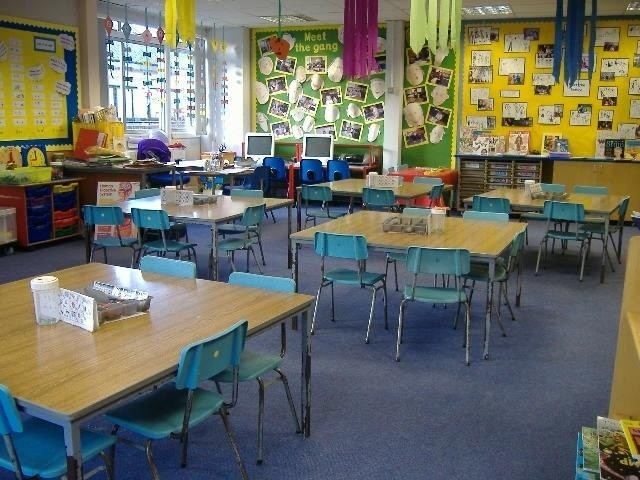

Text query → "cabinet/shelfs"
[601,235,640,422]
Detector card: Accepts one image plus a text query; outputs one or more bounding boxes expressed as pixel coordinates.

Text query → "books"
[575,416,640,479]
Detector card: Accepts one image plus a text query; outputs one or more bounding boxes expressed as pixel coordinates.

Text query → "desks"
[1,155,179,257]
[463,187,631,263]
[312,178,454,211]
[2,260,317,480]
[179,170,260,197]
[287,209,530,361]
[251,161,380,178]
[128,148,185,161]
[94,195,294,280]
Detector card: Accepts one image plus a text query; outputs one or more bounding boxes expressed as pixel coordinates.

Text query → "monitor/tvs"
[303,134,333,165]
[245,133,275,166]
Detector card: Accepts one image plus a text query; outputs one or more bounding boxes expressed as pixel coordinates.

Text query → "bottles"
[524,180,535,199]
[165,186,177,206]
[431,207,445,233]
[369,172,378,189]
[30,274,61,327]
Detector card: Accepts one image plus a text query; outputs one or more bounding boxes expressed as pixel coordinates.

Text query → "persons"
[342,127,353,138]
[613,147,623,160]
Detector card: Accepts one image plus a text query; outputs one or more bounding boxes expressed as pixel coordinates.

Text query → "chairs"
[428,183,444,208]
[207,202,267,282]
[262,157,290,200]
[309,230,387,342]
[472,195,512,215]
[83,202,143,268]
[534,202,591,281]
[519,183,566,246]
[565,185,607,249]
[134,187,191,261]
[126,206,201,277]
[101,317,250,480]
[0,385,118,479]
[326,159,352,182]
[299,158,325,185]
[411,177,443,209]
[226,164,276,225]
[393,246,475,365]
[137,256,199,279]
[381,208,448,301]
[208,271,303,467]
[298,184,351,249]
[207,189,265,266]
[362,187,394,212]
[578,192,631,273]
